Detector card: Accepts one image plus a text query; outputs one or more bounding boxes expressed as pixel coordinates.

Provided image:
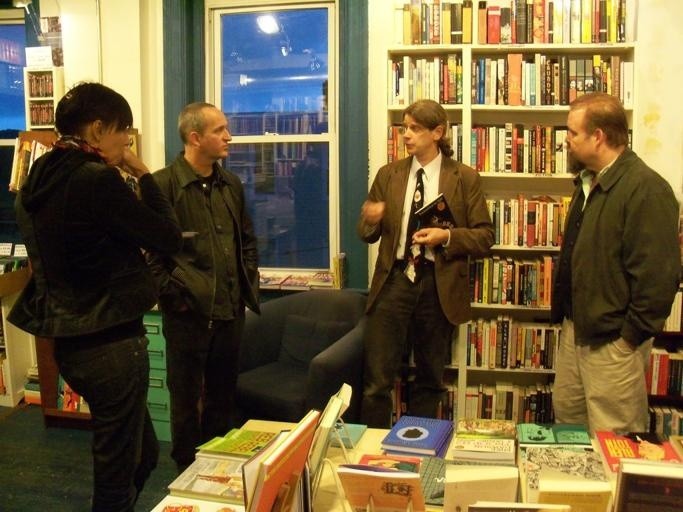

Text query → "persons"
[355,98,495,429]
[0,81,183,512]
[132,100,262,471]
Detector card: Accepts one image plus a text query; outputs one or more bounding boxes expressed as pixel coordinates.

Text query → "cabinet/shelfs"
[0,254,34,407]
[389,1,682,422]
[135,309,171,441]
[22,66,65,133]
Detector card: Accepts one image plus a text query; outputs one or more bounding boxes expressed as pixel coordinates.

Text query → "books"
[258,273,289,291]
[280,271,315,291]
[484,193,573,246]
[387,51,634,109]
[310,284,335,290]
[152,383,682,512]
[393,1,636,46]
[469,255,560,307]
[0,68,88,416]
[388,121,634,174]
[444,312,563,369]
[644,287,682,396]
[308,271,335,287]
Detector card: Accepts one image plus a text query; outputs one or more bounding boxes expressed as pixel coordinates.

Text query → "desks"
[153,422,682,512]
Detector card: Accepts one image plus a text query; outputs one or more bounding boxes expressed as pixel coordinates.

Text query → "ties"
[400,167,426,285]
